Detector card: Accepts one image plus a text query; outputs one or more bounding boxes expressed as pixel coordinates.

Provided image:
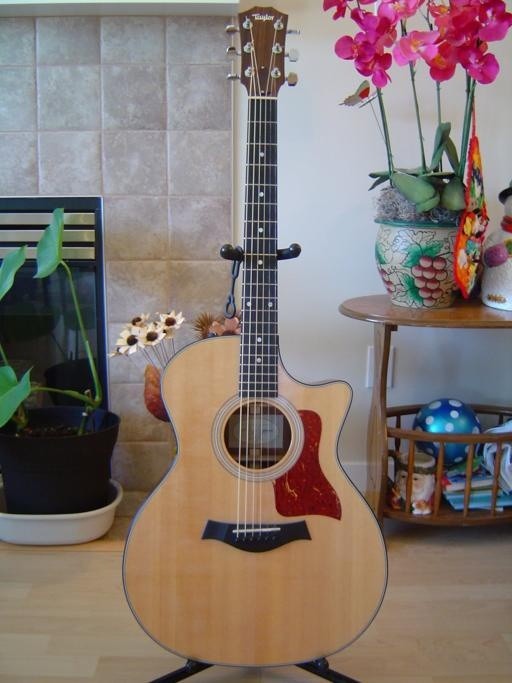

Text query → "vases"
[377,188,485,311]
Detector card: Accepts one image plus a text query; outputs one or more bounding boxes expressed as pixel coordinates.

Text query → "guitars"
[121,6,387,667]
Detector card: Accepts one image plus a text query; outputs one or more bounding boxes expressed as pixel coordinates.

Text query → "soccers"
[413,398,483,465]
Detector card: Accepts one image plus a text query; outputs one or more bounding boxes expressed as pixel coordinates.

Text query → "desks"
[338,287,512,531]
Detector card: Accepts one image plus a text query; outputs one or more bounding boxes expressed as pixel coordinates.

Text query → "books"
[443,457,512,510]
[479,506,503,512]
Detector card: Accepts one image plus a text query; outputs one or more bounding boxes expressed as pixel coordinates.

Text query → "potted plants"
[0,208,119,513]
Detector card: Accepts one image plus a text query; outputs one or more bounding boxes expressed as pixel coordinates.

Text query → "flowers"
[107,309,184,375]
[323,0,512,215]
